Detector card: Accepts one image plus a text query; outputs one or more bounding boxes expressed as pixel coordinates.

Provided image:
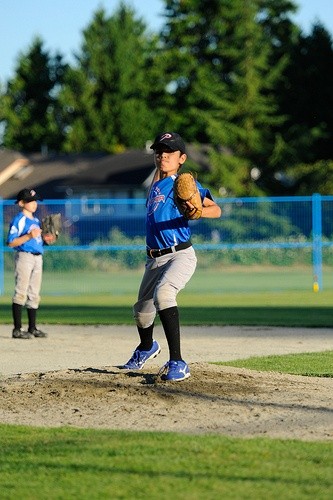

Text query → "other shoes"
[28,329,46,337]
[12,329,34,338]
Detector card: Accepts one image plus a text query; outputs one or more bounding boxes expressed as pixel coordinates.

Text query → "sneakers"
[158,360,191,381]
[123,339,161,370]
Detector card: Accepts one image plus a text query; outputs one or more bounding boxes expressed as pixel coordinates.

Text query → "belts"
[146,241,192,258]
[22,251,40,255]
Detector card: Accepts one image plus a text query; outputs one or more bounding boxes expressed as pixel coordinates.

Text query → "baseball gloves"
[41,214,62,245]
[173,173,203,220]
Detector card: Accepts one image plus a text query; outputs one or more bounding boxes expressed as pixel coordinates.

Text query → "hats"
[151,132,185,154]
[15,189,43,204]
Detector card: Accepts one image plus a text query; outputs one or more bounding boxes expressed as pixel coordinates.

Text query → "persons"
[7,189,61,339]
[124,132,222,381]
